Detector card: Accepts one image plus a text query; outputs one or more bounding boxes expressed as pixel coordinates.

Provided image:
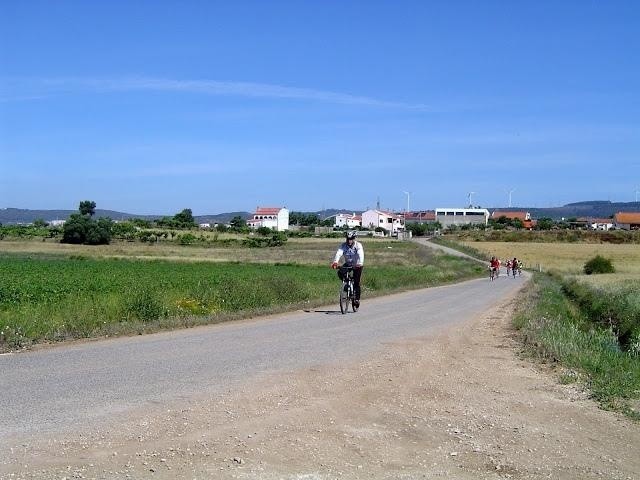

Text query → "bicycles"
[337,265,359,314]
[488,266,520,281]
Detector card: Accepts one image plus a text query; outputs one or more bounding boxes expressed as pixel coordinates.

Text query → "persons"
[489,256,524,279]
[331,230,364,309]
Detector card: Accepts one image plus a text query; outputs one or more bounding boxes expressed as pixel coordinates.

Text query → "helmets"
[346,232,356,239]
